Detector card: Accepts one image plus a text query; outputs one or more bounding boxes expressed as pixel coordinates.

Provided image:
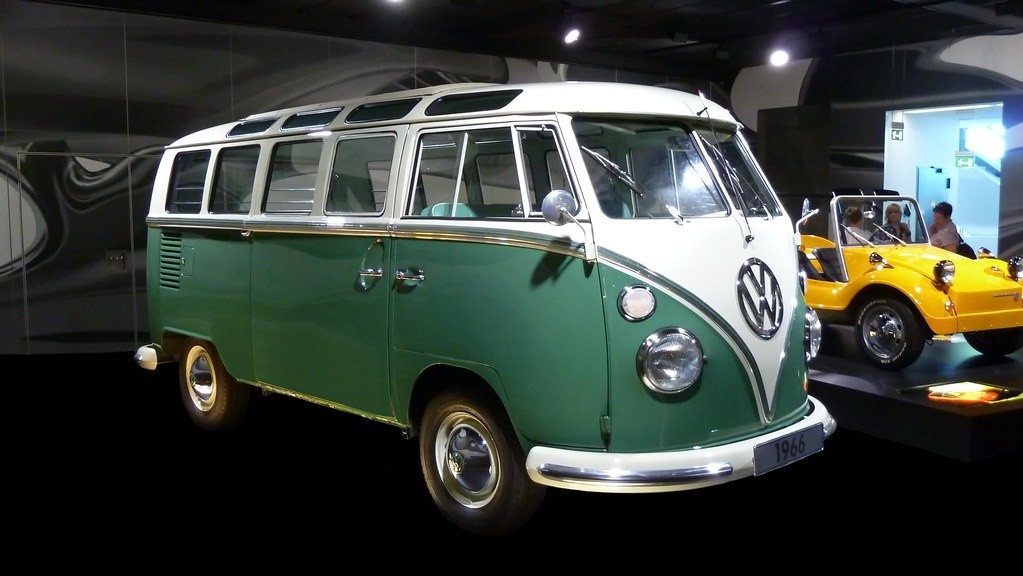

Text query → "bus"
[132,79,840,532]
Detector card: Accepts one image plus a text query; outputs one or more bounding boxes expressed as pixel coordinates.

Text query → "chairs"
[801,235,836,273]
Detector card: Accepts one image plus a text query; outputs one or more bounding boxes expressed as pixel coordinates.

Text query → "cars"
[795,187,1023,374]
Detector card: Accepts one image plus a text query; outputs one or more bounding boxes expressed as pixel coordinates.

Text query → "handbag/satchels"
[955,234,977,259]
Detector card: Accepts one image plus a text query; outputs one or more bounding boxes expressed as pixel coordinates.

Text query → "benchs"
[430,201,635,218]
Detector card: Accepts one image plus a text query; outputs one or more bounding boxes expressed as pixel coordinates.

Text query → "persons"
[928,202,958,253]
[842,202,913,244]
